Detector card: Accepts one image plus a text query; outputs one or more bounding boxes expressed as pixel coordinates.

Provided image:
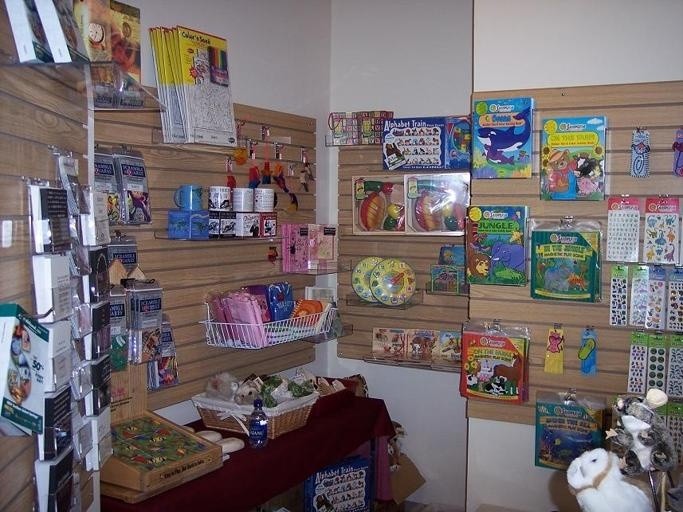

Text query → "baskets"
[199,309,339,349]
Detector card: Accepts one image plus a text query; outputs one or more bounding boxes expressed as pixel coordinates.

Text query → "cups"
[172,181,275,212]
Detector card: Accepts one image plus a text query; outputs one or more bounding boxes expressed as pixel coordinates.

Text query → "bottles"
[249,400,268,447]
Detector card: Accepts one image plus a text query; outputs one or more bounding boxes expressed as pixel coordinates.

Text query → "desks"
[99,375,396,512]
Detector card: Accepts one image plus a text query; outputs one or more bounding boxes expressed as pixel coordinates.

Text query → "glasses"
[195,403,313,439]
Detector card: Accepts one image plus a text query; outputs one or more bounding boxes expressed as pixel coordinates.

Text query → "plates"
[350,257,416,308]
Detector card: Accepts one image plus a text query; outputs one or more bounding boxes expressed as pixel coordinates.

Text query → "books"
[535,401,608,473]
[473,98,535,179]
[0,152,179,512]
[149,25,239,149]
[465,205,530,287]
[1,1,94,65]
[531,231,601,303]
[540,116,606,201]
[461,331,529,404]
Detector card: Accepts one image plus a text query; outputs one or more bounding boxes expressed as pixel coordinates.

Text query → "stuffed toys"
[203,371,262,407]
[567,388,678,512]
